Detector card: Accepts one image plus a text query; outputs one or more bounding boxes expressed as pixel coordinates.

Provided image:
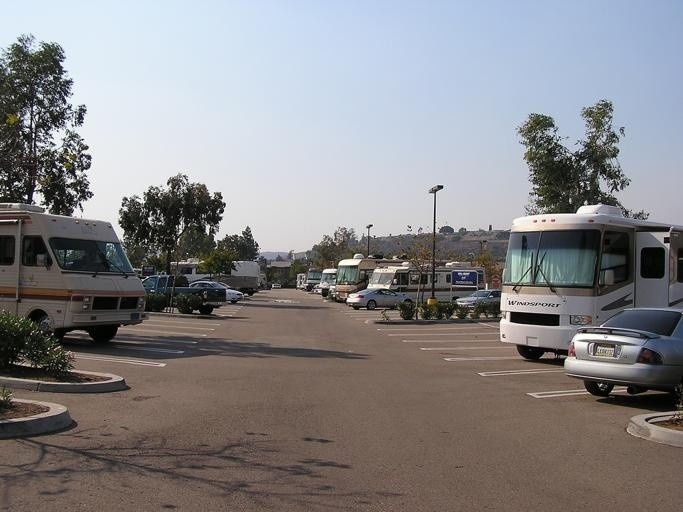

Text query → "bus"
[168,256,261,297]
[498,202,682,365]
[291,253,486,311]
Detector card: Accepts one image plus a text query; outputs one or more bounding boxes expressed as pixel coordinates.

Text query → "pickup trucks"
[140,274,227,318]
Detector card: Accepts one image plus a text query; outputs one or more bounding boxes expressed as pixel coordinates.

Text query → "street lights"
[364,223,373,256]
[425,183,445,318]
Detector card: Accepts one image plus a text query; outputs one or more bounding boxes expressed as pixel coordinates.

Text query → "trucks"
[0,200,155,348]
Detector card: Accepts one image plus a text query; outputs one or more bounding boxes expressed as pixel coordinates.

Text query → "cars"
[563,307,682,397]
[187,281,246,305]
[455,287,500,311]
[270,282,281,289]
[187,277,240,290]
